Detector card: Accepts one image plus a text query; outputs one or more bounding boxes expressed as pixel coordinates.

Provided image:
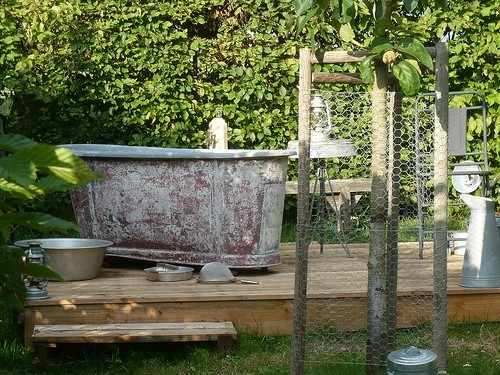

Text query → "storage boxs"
[453,233,468,256]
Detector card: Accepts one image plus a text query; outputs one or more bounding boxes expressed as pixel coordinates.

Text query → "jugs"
[459,193,500,287]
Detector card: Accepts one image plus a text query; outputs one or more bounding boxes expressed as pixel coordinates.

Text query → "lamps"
[310,91,332,142]
[23,241,51,301]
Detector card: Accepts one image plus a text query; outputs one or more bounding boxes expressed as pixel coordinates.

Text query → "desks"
[286,140,359,260]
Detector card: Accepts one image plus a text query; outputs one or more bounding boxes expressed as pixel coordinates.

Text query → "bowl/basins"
[14,238,114,281]
[144,266,194,281]
